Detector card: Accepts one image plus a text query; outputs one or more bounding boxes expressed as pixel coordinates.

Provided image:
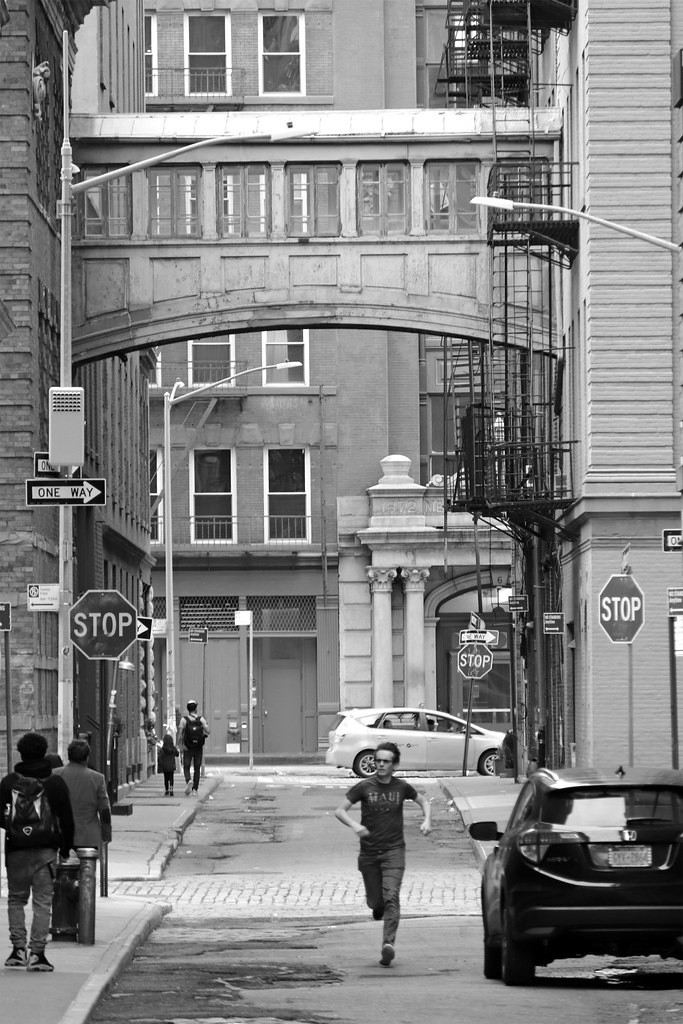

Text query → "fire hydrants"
[48,847,81,942]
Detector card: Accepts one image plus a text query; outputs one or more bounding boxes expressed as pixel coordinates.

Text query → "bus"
[458,708,510,732]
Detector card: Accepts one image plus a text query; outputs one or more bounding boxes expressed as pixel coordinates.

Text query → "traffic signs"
[662,529,683,553]
[24,477,107,506]
[458,629,501,646]
[34,452,82,478]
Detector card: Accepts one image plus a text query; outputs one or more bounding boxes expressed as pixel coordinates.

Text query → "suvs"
[468,769,683,986]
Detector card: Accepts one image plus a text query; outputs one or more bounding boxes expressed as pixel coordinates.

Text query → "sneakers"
[4,946,28,966]
[26,950,54,972]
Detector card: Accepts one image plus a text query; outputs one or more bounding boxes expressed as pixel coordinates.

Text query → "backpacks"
[5,771,60,847]
[184,715,208,753]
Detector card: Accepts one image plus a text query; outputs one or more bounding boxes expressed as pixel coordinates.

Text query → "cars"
[325,707,506,778]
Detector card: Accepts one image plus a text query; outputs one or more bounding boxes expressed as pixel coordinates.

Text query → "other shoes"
[170,788,173,796]
[379,944,395,965]
[372,908,384,920]
[192,790,197,796]
[185,780,194,795]
[165,791,169,796]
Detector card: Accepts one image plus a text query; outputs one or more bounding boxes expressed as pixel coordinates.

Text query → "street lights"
[164,362,302,778]
[47,126,320,762]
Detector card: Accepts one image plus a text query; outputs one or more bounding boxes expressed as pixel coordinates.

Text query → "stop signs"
[70,590,137,660]
[599,575,645,644]
[457,642,492,680]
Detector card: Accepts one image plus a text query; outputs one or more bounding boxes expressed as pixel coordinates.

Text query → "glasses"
[376,758,394,764]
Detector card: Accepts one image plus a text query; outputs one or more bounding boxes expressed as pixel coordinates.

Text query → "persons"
[159,734,179,796]
[179,703,210,795]
[334,743,432,965]
[48,740,112,852]
[0,733,74,971]
